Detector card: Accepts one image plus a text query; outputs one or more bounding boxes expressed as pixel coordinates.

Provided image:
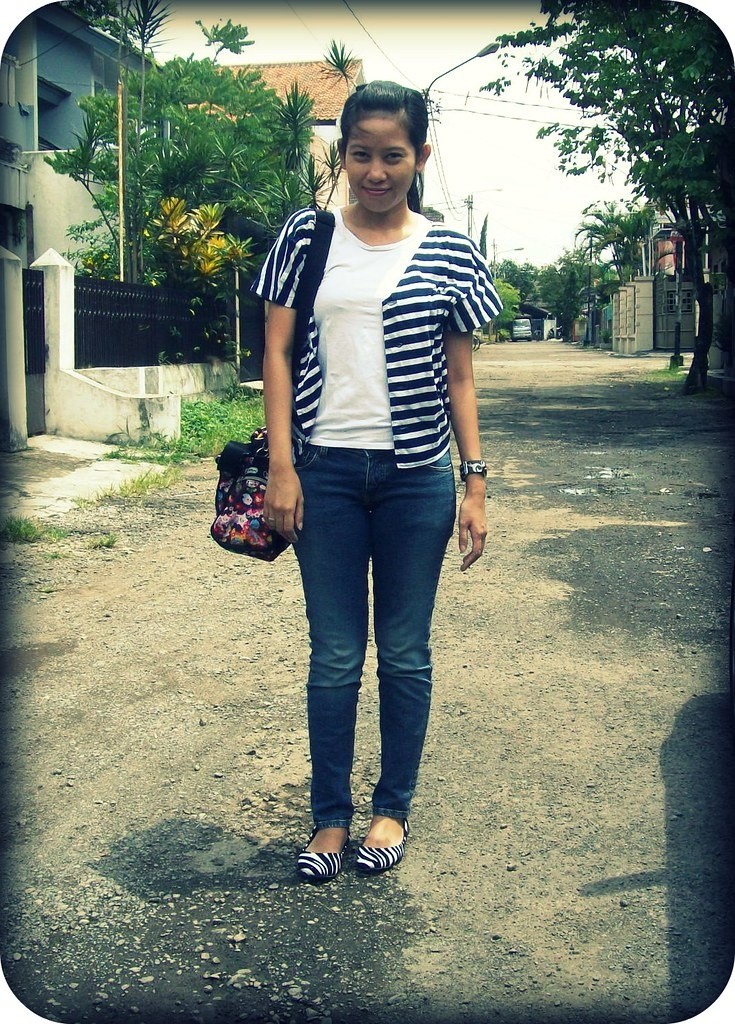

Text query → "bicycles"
[472,335,481,352]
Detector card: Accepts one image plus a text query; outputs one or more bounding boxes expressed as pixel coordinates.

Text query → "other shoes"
[356,810,409,875]
[297,818,351,882]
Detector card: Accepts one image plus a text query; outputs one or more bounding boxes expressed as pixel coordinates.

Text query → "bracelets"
[460,459,487,481]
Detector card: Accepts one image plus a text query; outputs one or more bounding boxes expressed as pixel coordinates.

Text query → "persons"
[249,80,503,881]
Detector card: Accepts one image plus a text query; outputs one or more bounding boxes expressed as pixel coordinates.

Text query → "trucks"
[511,319,533,341]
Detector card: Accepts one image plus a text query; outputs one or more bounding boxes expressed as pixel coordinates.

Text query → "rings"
[268,517,275,521]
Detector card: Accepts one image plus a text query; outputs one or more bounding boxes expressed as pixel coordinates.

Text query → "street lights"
[424,41,500,99]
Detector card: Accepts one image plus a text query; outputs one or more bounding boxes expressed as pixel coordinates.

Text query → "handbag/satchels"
[211,425,291,562]
